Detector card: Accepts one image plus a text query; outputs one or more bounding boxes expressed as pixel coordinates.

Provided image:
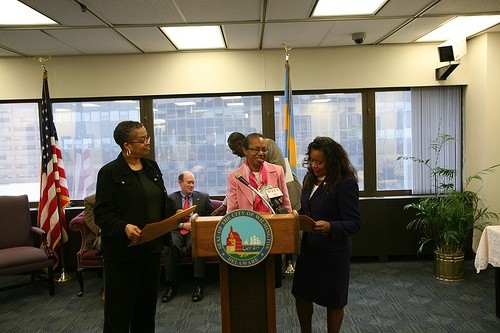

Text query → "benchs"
[69,199,224,297]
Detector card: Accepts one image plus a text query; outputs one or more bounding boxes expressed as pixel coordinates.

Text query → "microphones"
[235,173,275,214]
[265,184,287,214]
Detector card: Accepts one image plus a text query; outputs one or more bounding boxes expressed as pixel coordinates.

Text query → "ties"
[180,196,190,235]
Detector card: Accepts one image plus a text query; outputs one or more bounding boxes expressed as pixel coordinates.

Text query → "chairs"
[0,195,56,297]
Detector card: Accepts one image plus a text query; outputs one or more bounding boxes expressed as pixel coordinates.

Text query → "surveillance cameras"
[352,31,366,44]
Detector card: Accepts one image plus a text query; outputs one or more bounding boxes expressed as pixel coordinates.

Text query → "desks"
[474,225,500,319]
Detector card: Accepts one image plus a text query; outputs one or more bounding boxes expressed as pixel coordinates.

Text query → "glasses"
[245,147,269,155]
[130,136,151,145]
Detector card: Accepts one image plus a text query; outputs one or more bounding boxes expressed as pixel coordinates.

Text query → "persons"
[38,70,70,274]
[211,130,304,276]
[93,120,190,333]
[161,170,216,303]
[225,133,299,288]
[290,136,361,333]
[84,193,106,302]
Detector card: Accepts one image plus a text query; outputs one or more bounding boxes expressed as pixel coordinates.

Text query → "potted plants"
[396,118,500,282]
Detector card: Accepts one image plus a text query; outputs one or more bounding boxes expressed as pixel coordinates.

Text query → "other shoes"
[286,260,296,273]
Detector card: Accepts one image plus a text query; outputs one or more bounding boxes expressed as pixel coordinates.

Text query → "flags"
[281,62,302,178]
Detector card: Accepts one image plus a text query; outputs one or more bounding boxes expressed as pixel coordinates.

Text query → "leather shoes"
[161,286,176,302]
[192,285,202,302]
